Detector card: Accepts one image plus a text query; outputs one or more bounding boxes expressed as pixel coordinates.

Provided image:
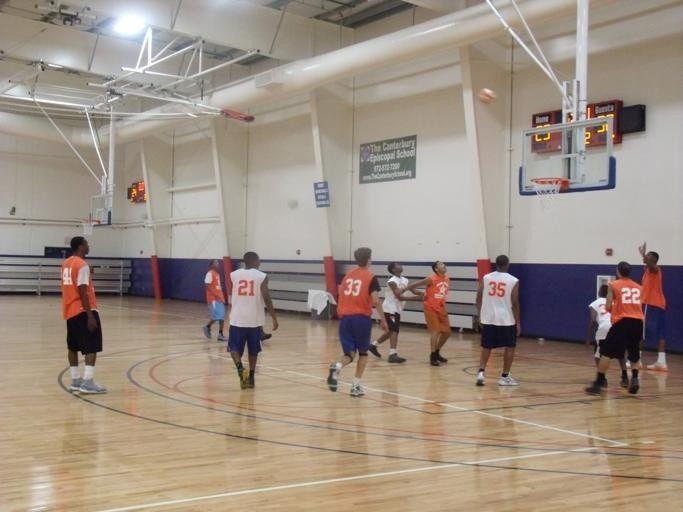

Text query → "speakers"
[618,104,646,133]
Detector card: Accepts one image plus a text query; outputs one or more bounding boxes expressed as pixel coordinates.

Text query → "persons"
[474,254,522,387]
[624,240,670,373]
[368,261,427,363]
[394,260,453,367]
[224,252,278,390]
[260,327,273,342]
[202,259,228,345]
[585,283,630,389]
[582,261,646,394]
[326,246,390,398]
[59,235,109,395]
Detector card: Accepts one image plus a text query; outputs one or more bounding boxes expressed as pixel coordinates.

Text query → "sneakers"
[368,344,381,357]
[71,377,83,391]
[626,360,643,368]
[498,372,520,386]
[437,353,447,362]
[431,355,439,366]
[620,379,629,387]
[476,372,486,385]
[203,325,211,340]
[217,333,227,341]
[328,362,338,392]
[351,384,366,396]
[629,377,638,393]
[249,377,254,388]
[647,362,668,371]
[584,388,601,396]
[389,353,406,362]
[593,379,607,387]
[79,379,107,394]
[239,368,249,389]
[259,332,271,341]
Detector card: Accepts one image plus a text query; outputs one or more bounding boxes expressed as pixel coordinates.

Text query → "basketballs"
[477,88,497,104]
